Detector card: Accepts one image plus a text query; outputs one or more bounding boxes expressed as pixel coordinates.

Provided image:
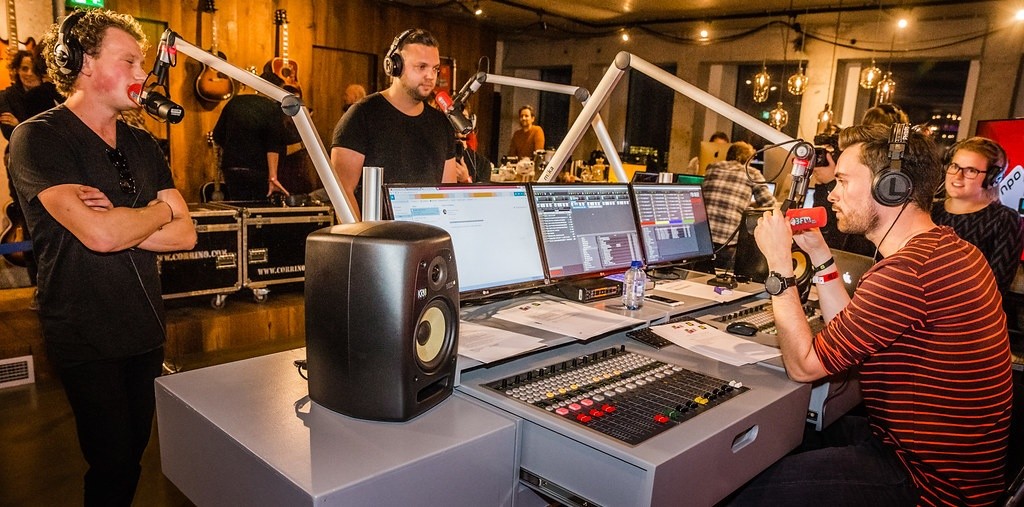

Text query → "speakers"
[304,221,460,422]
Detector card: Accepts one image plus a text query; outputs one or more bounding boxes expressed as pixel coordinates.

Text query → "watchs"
[764,271,797,296]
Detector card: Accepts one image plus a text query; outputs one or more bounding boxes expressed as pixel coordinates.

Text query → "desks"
[154,270,827,507]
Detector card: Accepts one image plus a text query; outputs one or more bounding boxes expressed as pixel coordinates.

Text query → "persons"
[342,84,367,113]
[508,105,545,161]
[730,123,1013,507]
[811,103,909,258]
[687,131,729,176]
[275,85,314,194]
[0,50,57,200]
[454,127,492,184]
[693,143,781,277]
[213,72,290,196]
[930,136,1024,301]
[331,28,457,225]
[9,9,197,507]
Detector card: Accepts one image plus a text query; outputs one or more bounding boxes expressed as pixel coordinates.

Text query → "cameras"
[810,132,844,166]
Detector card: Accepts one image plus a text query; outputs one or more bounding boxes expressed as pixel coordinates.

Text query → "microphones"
[779,198,828,229]
[435,91,474,135]
[128,84,184,125]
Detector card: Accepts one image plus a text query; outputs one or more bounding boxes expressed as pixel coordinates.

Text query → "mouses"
[727,321,758,336]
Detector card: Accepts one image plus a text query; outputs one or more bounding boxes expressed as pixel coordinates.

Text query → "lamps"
[751,13,896,133]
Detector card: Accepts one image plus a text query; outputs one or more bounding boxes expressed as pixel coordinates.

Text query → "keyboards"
[626,315,718,350]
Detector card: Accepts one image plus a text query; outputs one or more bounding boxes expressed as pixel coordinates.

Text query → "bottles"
[623,261,646,310]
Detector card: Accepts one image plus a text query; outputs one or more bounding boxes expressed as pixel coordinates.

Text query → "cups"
[785,205,829,232]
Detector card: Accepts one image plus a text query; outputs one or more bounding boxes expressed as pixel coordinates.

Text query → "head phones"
[53,11,87,76]
[270,192,308,206]
[942,138,1009,189]
[384,29,419,77]
[871,123,914,207]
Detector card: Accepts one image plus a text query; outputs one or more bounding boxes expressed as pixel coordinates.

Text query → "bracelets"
[813,256,834,272]
[812,270,839,284]
[155,199,174,231]
[463,176,472,183]
[268,177,277,182]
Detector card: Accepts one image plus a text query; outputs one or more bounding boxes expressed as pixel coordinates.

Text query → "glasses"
[944,162,988,180]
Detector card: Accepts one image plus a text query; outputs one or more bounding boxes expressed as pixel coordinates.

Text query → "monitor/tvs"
[676,174,705,187]
[381,182,548,302]
[750,180,777,203]
[629,171,660,183]
[528,182,646,283]
[628,182,716,269]
[975,117,1024,260]
